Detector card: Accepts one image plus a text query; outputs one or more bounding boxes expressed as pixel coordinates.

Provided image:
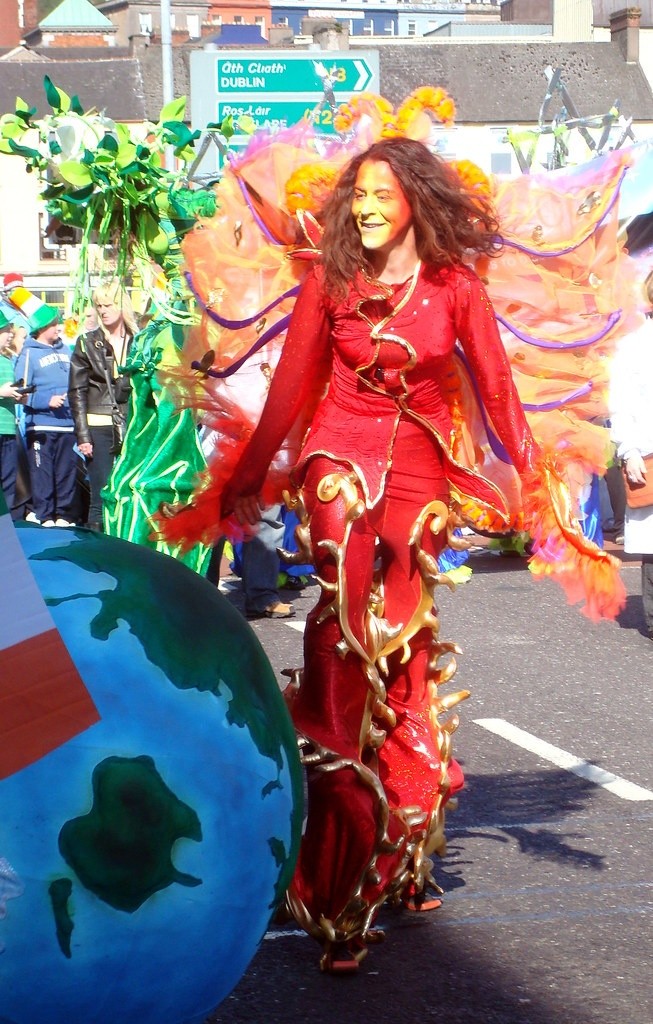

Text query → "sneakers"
[244,600,296,618]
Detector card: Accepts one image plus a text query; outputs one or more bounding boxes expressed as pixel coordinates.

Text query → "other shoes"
[319,934,370,974]
[401,887,442,911]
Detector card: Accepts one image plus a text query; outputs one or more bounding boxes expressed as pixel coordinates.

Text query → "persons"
[0,268,652,641]
[219,137,556,970]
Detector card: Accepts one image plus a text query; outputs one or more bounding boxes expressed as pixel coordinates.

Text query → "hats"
[8,286,60,334]
[3,273,24,291]
[0,310,9,330]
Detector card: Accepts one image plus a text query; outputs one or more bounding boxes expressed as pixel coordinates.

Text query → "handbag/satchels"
[110,403,129,454]
